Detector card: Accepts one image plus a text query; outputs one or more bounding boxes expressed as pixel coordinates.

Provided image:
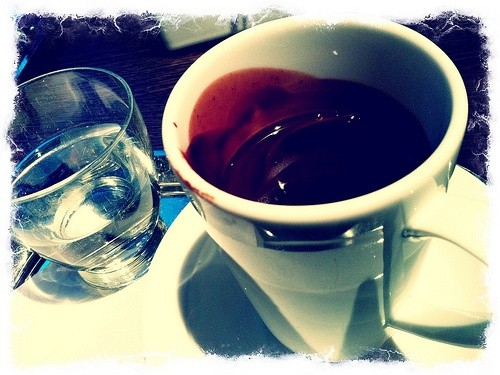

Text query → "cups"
[162,18,488,361]
[11,66,167,288]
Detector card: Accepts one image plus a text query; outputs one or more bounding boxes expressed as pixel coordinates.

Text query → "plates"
[153,165,487,360]
